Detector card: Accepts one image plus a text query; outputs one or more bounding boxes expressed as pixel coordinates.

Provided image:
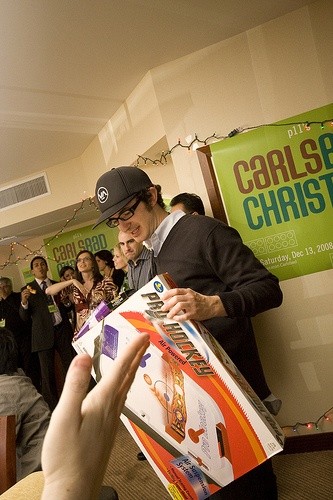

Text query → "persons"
[0,326,52,469]
[20,256,76,413]
[169,193,205,215]
[59,266,76,281]
[112,231,151,291]
[0,277,21,350]
[94,250,125,287]
[41,332,151,500]
[95,166,283,500]
[45,250,118,335]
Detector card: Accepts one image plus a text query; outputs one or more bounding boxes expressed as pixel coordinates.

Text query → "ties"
[41,281,56,326]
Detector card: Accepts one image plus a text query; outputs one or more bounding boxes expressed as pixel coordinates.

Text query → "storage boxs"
[72,273,285,500]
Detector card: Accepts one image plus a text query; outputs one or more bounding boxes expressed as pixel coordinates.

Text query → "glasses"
[106,190,146,228]
[76,257,92,263]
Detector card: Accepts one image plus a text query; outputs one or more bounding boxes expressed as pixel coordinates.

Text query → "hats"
[92,166,154,230]
